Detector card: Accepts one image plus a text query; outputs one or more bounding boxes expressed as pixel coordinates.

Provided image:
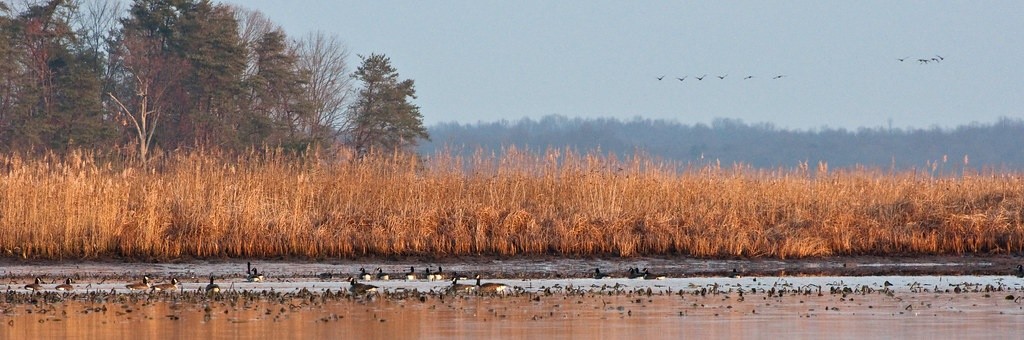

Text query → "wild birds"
[657,73,787,81]
[1,260,1024,326]
[896,54,949,64]
